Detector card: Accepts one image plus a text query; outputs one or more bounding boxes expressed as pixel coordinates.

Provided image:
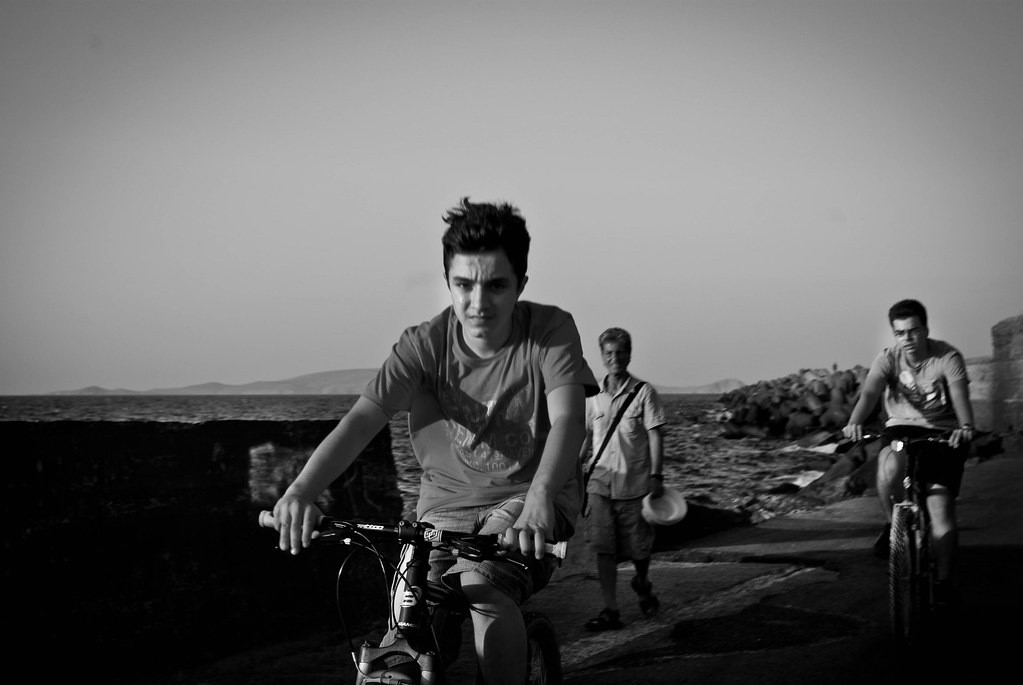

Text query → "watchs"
[646,474,664,483]
[960,423,974,430]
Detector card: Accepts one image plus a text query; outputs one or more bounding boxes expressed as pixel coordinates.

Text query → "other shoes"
[873,520,892,557]
[586,607,622,632]
[630,574,658,618]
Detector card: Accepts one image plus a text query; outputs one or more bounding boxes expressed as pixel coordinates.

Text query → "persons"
[841,300,975,607]
[577,328,668,632]
[271,201,600,685]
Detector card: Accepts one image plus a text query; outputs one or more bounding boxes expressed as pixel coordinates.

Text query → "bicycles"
[842,425,974,638]
[258,510,568,685]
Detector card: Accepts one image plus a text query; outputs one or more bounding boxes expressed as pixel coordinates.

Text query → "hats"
[643,488,689,523]
